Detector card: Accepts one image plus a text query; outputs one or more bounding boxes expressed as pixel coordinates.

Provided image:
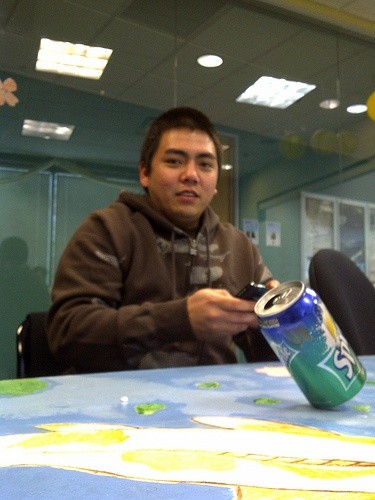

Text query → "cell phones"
[235,281,270,301]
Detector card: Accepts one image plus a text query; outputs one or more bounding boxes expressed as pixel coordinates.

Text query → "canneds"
[254,280,367,409]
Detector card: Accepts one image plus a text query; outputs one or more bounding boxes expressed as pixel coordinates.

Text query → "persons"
[43,107,284,374]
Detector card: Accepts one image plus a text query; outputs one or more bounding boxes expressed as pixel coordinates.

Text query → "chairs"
[311,249,375,357]
[18,313,55,377]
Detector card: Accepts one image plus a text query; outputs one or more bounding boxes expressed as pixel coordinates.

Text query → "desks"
[0,356,374,500]
